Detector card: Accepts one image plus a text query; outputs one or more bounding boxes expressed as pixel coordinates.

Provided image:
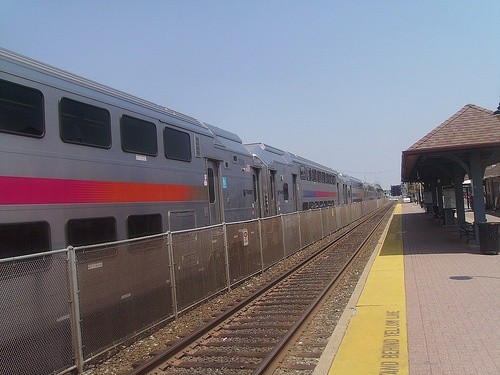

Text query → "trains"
[0,44,387,347]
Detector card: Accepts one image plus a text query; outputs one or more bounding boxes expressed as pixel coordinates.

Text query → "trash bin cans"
[444,209,455,225]
[477,222,500,255]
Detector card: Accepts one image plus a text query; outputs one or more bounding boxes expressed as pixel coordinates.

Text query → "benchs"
[456,217,487,244]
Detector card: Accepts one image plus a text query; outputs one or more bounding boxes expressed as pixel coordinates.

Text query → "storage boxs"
[476,222,500,255]
